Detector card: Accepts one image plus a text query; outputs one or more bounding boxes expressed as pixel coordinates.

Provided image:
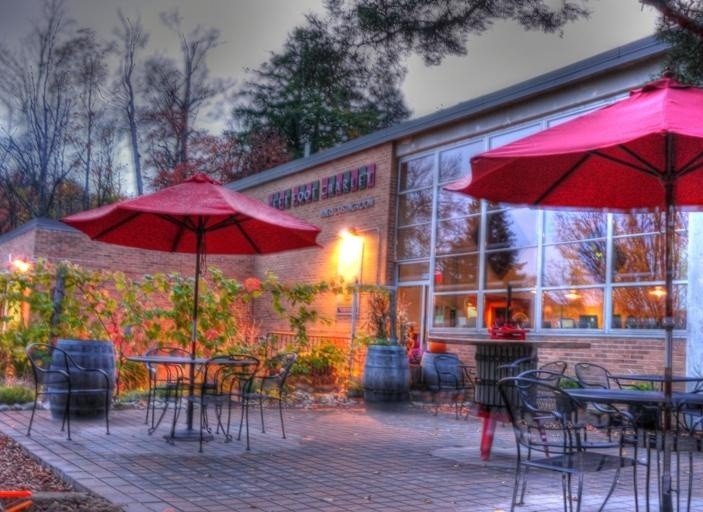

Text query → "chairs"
[143,345,297,449]
[24,343,111,442]
[429,354,702,512]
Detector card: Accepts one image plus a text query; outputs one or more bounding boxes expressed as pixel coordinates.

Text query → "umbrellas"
[58,170,323,432]
[441,72,703,511]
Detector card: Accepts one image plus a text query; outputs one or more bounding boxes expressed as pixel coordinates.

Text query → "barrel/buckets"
[429,340,447,354]
[419,354,462,396]
[47,338,114,422]
[474,343,538,406]
[364,344,411,405]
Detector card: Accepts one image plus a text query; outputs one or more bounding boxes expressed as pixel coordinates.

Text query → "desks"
[126,355,255,443]
